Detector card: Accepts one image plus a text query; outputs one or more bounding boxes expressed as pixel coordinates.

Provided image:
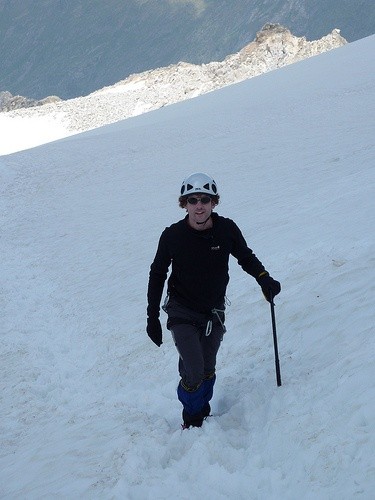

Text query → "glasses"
[187,197,212,205]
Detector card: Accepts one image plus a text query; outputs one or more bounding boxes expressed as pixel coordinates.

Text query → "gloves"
[256,272,281,306]
[146,318,163,347]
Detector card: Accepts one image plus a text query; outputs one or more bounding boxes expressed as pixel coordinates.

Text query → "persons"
[146,173,281,430]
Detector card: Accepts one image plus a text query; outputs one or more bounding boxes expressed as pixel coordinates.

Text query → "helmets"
[179,173,218,196]
